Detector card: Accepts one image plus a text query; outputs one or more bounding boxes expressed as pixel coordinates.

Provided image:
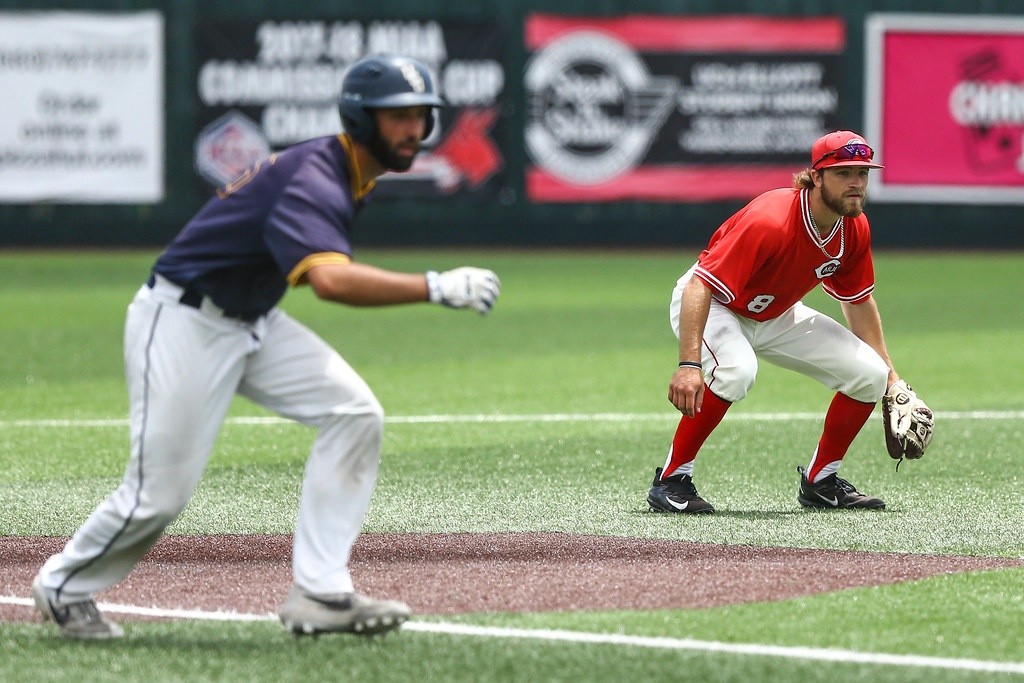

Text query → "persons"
[646,130,934,516]
[31,54,501,643]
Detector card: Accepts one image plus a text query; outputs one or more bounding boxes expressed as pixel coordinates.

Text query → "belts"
[148,275,239,319]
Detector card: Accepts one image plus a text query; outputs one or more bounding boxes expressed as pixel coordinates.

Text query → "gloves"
[426,267,500,316]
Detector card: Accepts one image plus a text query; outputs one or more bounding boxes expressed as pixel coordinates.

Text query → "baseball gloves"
[882,378,937,461]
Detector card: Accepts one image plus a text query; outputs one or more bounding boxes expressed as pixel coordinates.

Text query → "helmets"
[810,130,885,172]
[337,53,444,143]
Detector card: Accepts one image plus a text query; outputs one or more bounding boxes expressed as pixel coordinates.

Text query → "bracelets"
[678,362,702,369]
[679,365,701,370]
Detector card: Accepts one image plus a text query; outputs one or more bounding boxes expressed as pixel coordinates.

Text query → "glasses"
[812,143,874,169]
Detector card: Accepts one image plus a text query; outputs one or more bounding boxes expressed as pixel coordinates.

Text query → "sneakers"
[796,465,886,510]
[278,585,414,636]
[647,466,715,515]
[32,575,124,640]
